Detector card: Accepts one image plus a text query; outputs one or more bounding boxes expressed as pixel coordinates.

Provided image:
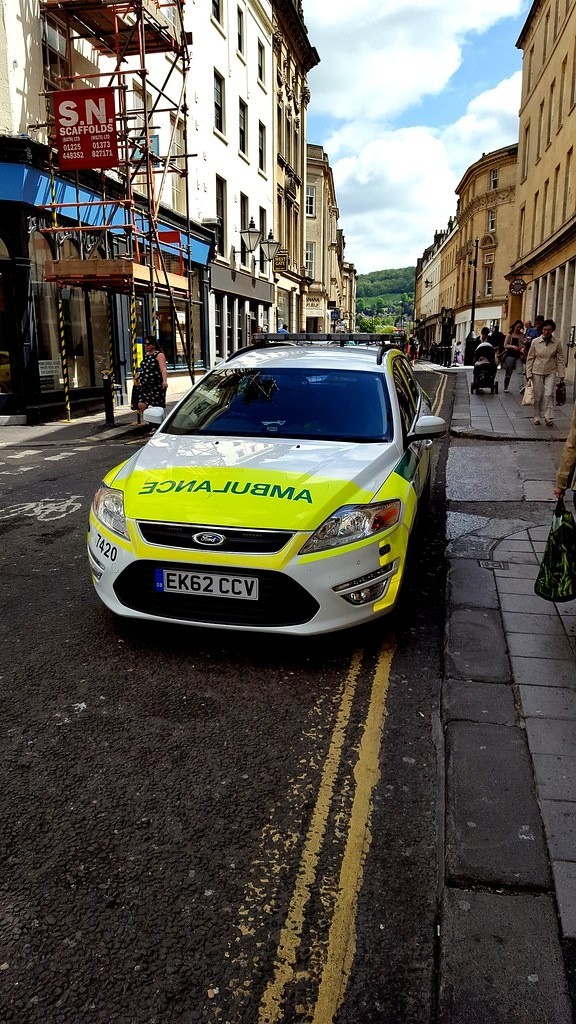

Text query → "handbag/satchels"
[496,350,508,363]
[556,380,566,406]
[521,379,535,406]
[534,497,576,602]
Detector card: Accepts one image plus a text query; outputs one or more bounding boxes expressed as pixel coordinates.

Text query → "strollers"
[470,341,499,395]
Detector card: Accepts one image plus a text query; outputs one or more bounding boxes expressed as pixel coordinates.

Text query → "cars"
[86,331,447,639]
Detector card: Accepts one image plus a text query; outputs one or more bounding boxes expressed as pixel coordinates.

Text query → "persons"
[135,336,167,435]
[526,319,566,426]
[554,398,576,513]
[252,315,546,393]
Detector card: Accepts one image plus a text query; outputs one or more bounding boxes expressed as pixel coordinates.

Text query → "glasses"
[143,343,151,346]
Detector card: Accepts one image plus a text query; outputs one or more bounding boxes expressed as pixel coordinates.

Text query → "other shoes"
[149,429,157,435]
[545,419,553,426]
[503,388,509,393]
[533,416,540,425]
[520,386,526,394]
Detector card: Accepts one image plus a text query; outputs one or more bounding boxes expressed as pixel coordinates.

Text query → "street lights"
[464,235,480,365]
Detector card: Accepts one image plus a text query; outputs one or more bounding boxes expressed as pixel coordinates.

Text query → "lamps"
[424,278,432,288]
[253,228,282,269]
[232,216,265,261]
